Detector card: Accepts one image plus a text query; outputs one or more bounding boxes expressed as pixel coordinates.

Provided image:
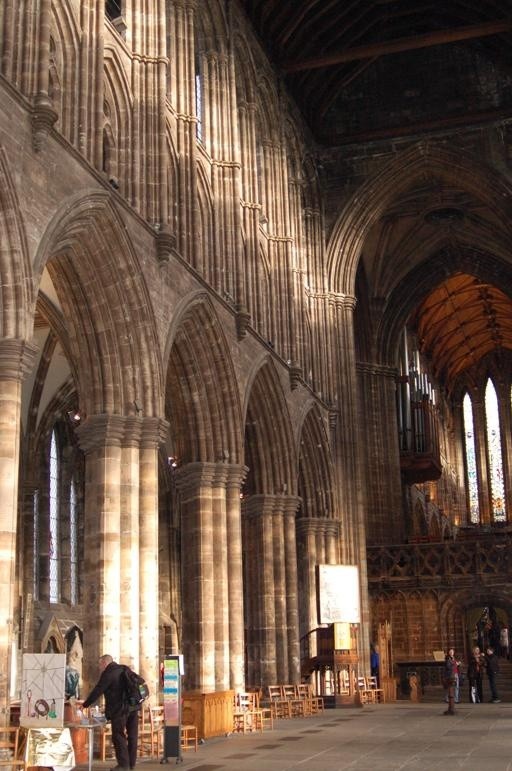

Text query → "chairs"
[331,677,385,706]
[97,703,198,764]
[234,684,324,738]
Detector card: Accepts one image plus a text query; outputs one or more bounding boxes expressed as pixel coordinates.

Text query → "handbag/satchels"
[471,687,480,703]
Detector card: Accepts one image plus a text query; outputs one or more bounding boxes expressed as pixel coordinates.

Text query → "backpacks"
[109,665,149,705]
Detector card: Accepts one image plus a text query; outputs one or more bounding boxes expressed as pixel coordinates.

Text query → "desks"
[182,689,235,744]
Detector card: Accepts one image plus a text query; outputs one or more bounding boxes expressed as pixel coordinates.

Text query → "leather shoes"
[110,765,135,771]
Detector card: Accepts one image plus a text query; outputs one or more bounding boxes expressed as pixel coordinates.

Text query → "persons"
[371,643,379,676]
[446,646,501,703]
[79,655,139,771]
